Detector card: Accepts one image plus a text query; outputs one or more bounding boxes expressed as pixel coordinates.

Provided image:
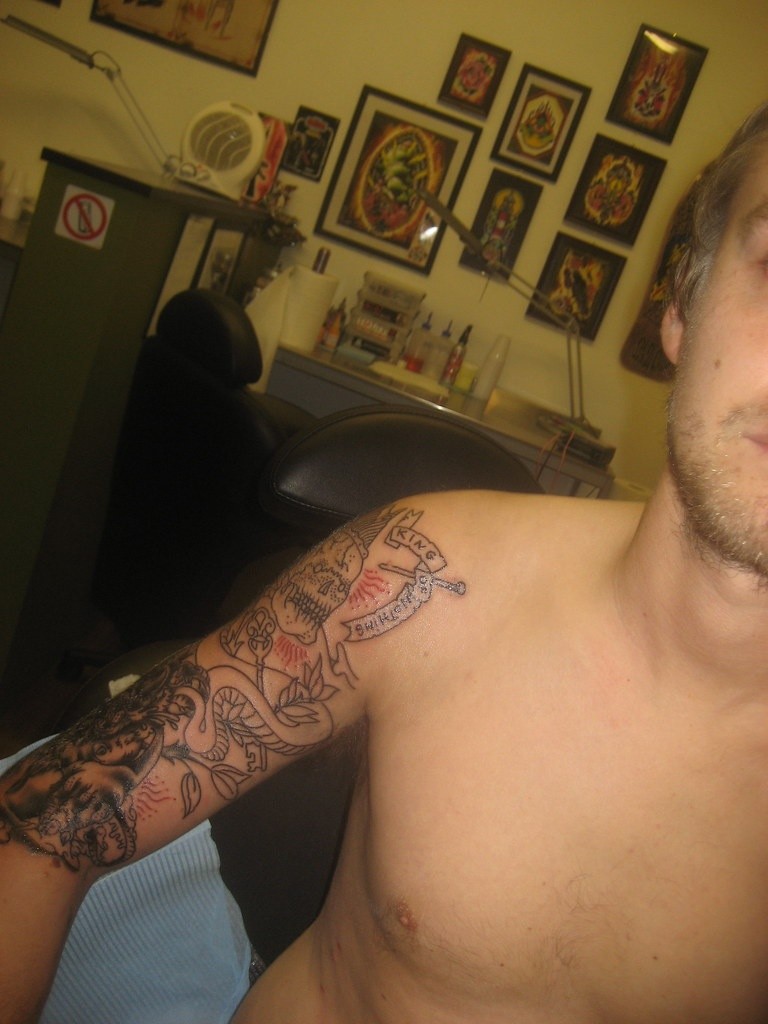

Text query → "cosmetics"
[314,298,514,402]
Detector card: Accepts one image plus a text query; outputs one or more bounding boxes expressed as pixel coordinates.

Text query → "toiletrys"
[467,334,510,403]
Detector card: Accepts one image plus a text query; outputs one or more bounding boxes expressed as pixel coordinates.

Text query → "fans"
[171,99,270,204]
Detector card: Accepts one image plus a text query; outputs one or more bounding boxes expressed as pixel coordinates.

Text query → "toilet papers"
[243,265,339,395]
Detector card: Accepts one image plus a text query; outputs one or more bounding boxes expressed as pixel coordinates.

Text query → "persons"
[0,97,768,1023]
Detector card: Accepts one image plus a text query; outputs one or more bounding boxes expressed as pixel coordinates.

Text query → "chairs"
[85,287,324,662]
[41,402,552,976]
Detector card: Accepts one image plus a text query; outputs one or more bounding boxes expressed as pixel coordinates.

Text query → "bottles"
[421,320,454,381]
[405,313,435,373]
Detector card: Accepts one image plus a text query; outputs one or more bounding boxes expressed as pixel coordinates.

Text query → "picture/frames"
[435,32,512,119]
[279,105,341,183]
[313,84,484,276]
[457,167,544,284]
[88,0,281,79]
[604,22,710,146]
[489,62,592,186]
[564,134,667,246]
[525,231,628,342]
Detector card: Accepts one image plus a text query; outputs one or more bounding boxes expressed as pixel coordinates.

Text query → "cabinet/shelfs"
[264,342,618,501]
